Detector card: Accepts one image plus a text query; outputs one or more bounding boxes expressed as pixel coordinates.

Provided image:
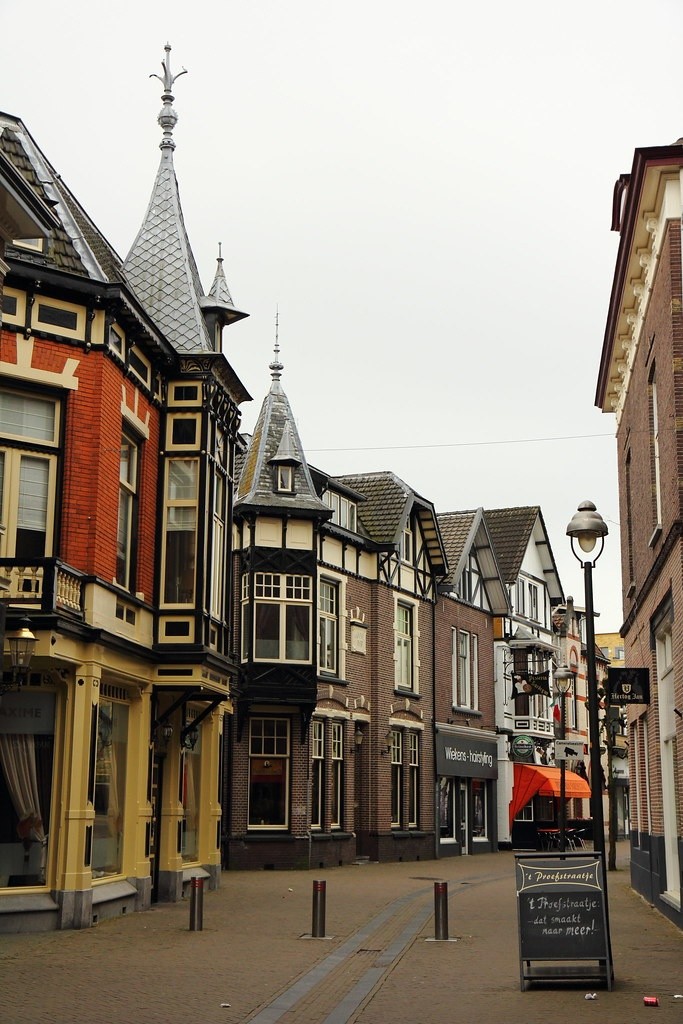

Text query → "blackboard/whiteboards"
[515,851,609,961]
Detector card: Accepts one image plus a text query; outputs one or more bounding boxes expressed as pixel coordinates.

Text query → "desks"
[536,828,561,851]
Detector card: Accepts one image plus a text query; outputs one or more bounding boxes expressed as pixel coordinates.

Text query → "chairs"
[536,826,587,852]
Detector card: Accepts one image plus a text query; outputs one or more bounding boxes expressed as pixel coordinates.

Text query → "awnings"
[509,763,592,834]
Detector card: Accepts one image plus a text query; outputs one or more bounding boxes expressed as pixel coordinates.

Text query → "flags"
[552,698,560,722]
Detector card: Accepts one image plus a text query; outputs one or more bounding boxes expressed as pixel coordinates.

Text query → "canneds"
[643,996,659,1006]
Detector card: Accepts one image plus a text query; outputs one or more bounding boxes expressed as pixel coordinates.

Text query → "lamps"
[448,718,470,727]
[154,718,174,754]
[481,725,500,733]
[0,609,40,698]
[180,724,198,751]
[381,729,395,756]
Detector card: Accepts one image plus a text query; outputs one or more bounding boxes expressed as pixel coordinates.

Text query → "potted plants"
[350,726,364,754]
